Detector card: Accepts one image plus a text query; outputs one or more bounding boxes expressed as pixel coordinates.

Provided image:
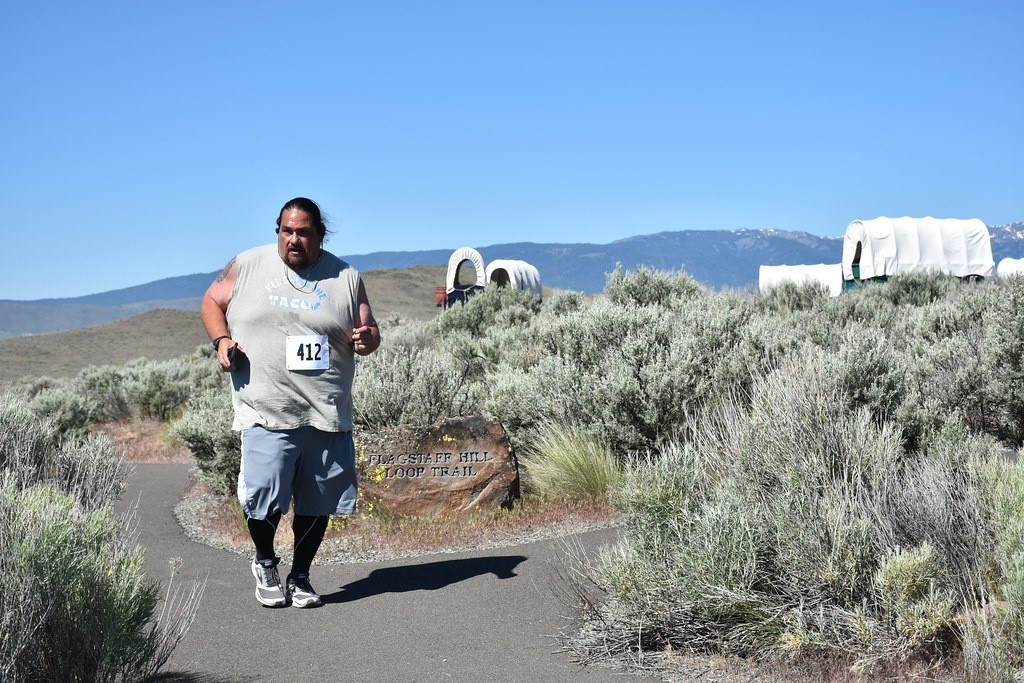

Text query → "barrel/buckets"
[435,287,446,306]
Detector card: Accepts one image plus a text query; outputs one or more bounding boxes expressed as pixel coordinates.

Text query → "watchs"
[213,336,229,352]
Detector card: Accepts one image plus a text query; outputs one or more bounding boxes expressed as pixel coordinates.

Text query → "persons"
[201,197,381,608]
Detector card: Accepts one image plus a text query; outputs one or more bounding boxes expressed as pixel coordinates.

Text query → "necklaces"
[284,263,313,290]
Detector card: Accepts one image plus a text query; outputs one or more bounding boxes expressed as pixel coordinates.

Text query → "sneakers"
[285,572,321,607]
[252,557,286,608]
